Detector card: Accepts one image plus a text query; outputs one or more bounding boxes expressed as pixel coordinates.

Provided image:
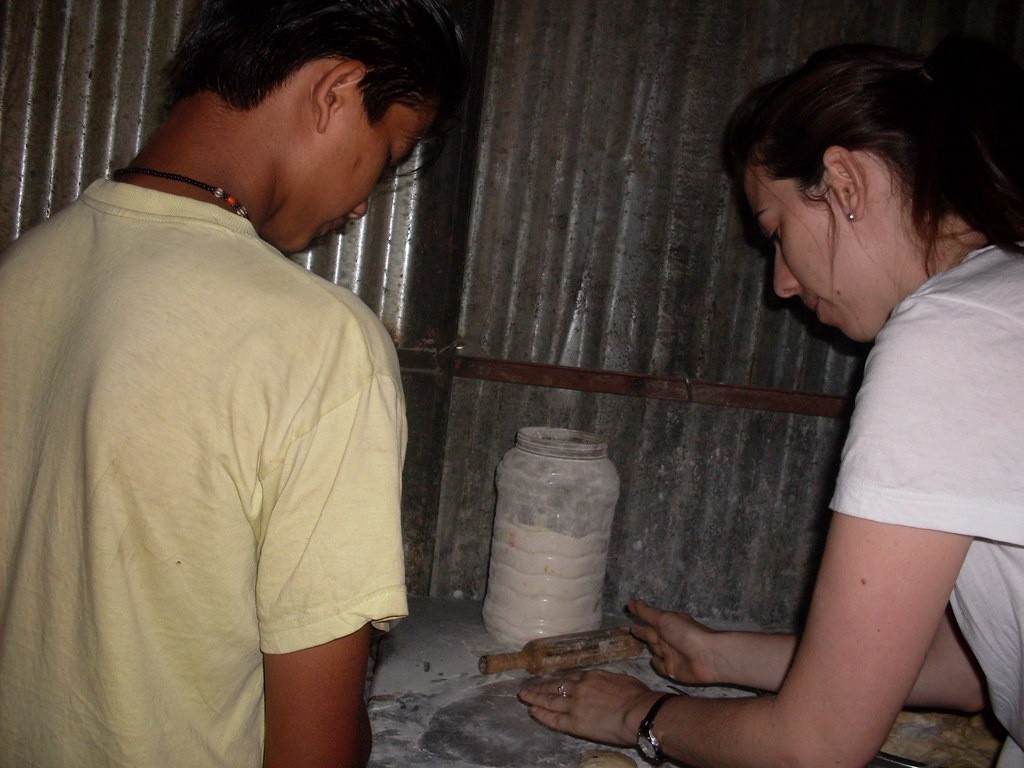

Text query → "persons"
[520,47,1024,768]
[0,1,468,767]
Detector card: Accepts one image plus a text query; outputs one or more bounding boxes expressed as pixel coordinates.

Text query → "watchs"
[637,686,689,766]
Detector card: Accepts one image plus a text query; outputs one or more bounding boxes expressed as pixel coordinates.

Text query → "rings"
[558,680,568,698]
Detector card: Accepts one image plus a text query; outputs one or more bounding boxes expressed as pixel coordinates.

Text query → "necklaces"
[113,168,250,219]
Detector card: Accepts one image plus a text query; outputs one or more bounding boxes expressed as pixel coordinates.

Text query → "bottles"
[480,427,621,651]
